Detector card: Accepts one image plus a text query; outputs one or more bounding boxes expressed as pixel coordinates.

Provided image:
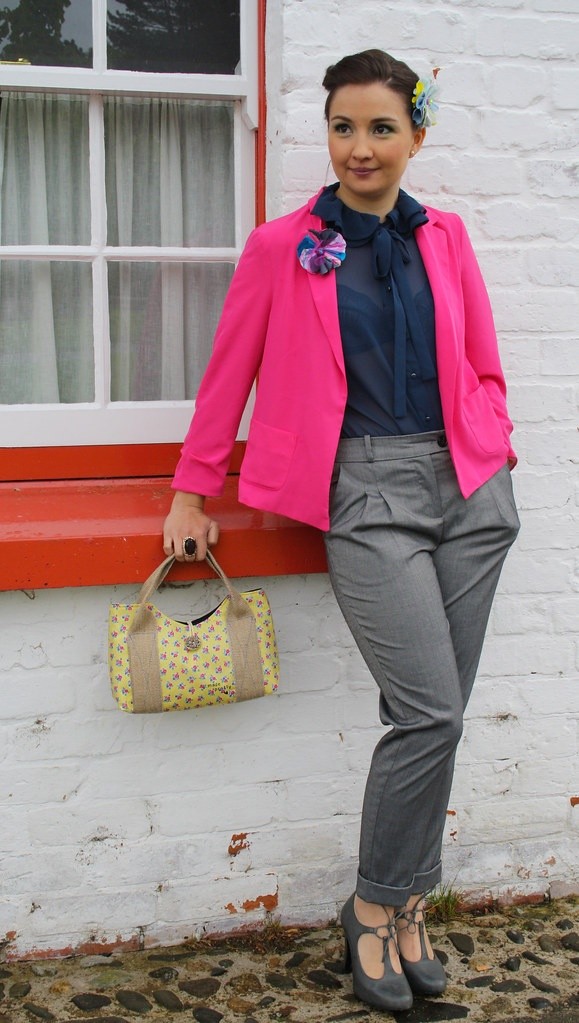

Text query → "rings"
[183,536,196,557]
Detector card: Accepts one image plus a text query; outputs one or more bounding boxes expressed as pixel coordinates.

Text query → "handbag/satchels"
[108,548,278,714]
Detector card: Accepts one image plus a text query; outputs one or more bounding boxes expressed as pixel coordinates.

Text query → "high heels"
[397,885,446,993]
[342,890,414,1010]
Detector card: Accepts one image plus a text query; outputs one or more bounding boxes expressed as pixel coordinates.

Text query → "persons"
[163,51,521,1015]
